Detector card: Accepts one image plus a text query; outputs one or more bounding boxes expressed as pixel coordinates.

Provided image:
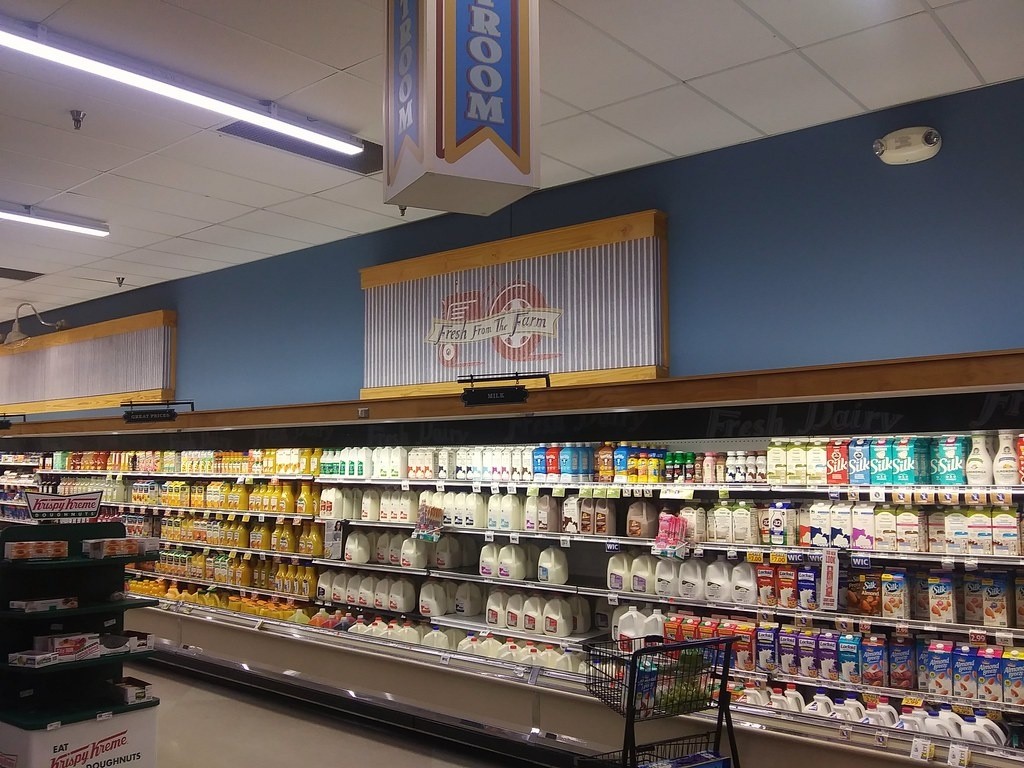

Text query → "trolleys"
[575,634,743,768]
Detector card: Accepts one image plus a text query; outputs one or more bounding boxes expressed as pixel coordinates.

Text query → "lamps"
[1,14,365,155]
[0,198,111,240]
[4,301,71,349]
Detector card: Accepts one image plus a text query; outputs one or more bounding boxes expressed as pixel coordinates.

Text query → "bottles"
[130,420,1024,750]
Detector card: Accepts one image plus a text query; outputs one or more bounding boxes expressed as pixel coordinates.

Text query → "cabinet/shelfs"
[2,427,1024,768]
[0,515,159,768]
